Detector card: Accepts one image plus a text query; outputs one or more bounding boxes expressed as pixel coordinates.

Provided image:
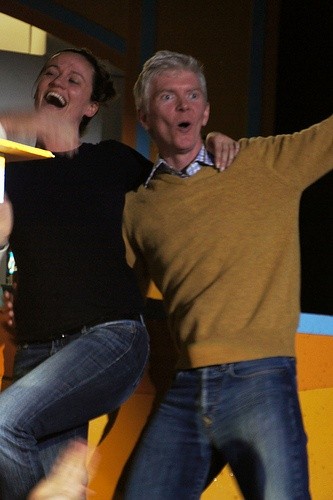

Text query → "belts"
[20,313,140,345]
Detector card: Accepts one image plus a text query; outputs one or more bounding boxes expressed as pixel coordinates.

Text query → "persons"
[109,50,333,500]
[0,50,234,500]
